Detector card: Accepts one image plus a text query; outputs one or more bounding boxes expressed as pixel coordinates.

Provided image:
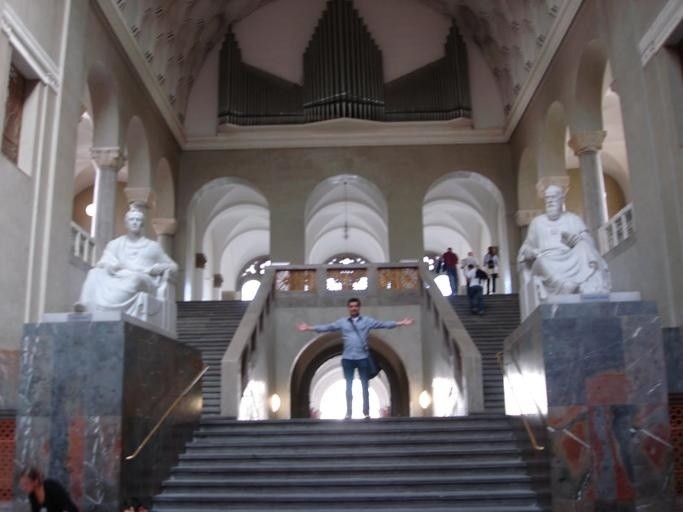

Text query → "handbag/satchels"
[366,354,382,380]
[476,269,488,279]
[436,261,448,275]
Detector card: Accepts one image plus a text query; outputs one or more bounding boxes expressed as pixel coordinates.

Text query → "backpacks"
[488,260,495,268]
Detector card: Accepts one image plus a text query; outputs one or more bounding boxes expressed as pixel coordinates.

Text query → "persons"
[74,210,179,312]
[439,247,500,316]
[19,465,80,512]
[297,298,415,419]
[518,185,612,295]
